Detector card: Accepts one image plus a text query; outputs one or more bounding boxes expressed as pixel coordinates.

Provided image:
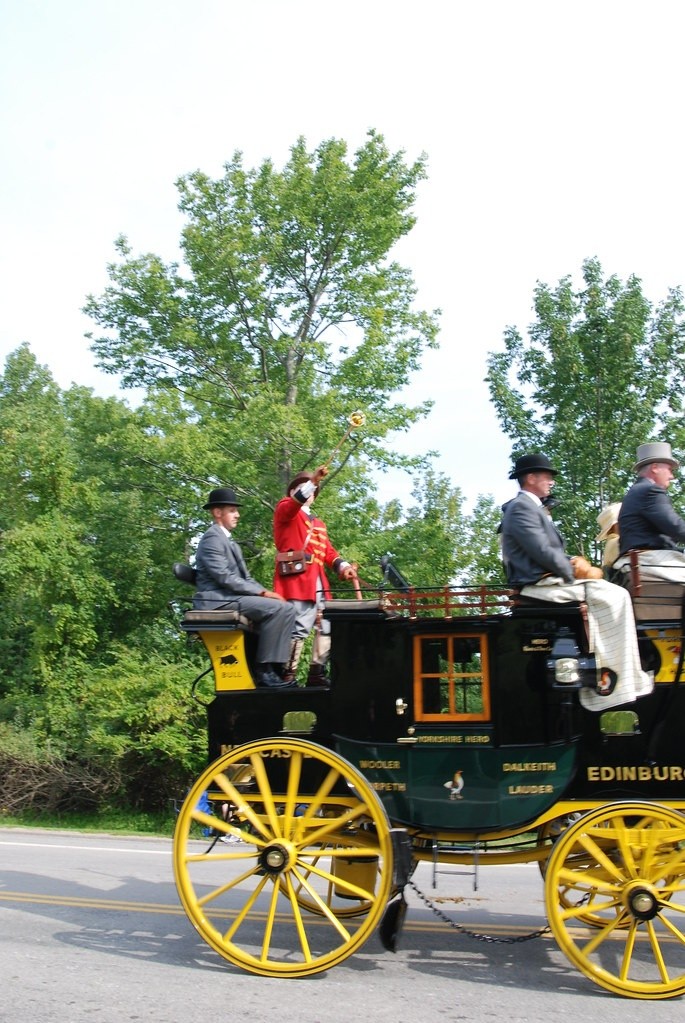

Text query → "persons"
[496,454,653,712]
[272,465,357,688]
[195,489,297,688]
[219,804,242,843]
[594,442,685,586]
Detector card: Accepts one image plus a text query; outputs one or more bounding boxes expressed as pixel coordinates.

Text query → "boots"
[284,670,301,687]
[306,664,331,689]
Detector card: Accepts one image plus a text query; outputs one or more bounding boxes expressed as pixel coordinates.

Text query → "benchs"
[603,545,684,622]
[509,589,587,620]
[314,554,414,622]
[172,563,259,636]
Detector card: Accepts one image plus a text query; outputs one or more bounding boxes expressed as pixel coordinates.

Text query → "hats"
[496,493,563,534]
[509,454,560,478]
[632,443,679,470]
[595,503,622,540]
[202,488,244,509]
[287,471,319,499]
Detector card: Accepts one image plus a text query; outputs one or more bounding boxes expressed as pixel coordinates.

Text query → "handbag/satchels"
[277,551,305,576]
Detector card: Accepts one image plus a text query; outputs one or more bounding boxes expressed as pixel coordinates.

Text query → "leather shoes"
[257,673,297,688]
